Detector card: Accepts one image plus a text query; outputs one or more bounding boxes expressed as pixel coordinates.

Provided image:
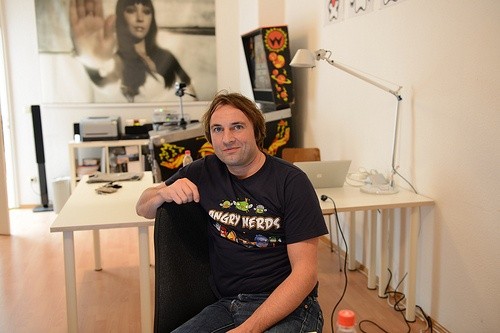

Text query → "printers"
[80,116,122,142]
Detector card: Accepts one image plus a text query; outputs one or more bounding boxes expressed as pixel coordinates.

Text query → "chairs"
[282,148,320,163]
[154,201,240,333]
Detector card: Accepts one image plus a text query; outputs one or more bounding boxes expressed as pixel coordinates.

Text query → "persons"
[135,92,329,333]
[67,1,201,106]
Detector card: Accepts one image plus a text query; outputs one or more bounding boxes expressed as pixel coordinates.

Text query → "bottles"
[337,310,356,332]
[183,150,193,167]
[152,110,177,130]
[133,119,141,127]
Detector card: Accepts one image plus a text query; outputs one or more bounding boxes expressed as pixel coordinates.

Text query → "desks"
[51,173,435,333]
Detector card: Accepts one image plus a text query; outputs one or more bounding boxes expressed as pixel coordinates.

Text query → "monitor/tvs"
[254,34,272,92]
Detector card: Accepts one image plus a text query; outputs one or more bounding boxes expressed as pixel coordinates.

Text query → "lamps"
[289,48,402,195]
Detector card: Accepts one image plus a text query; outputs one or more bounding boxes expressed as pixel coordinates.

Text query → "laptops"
[293,160,352,189]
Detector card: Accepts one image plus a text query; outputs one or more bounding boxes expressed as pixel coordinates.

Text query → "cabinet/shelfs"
[70,139,151,193]
